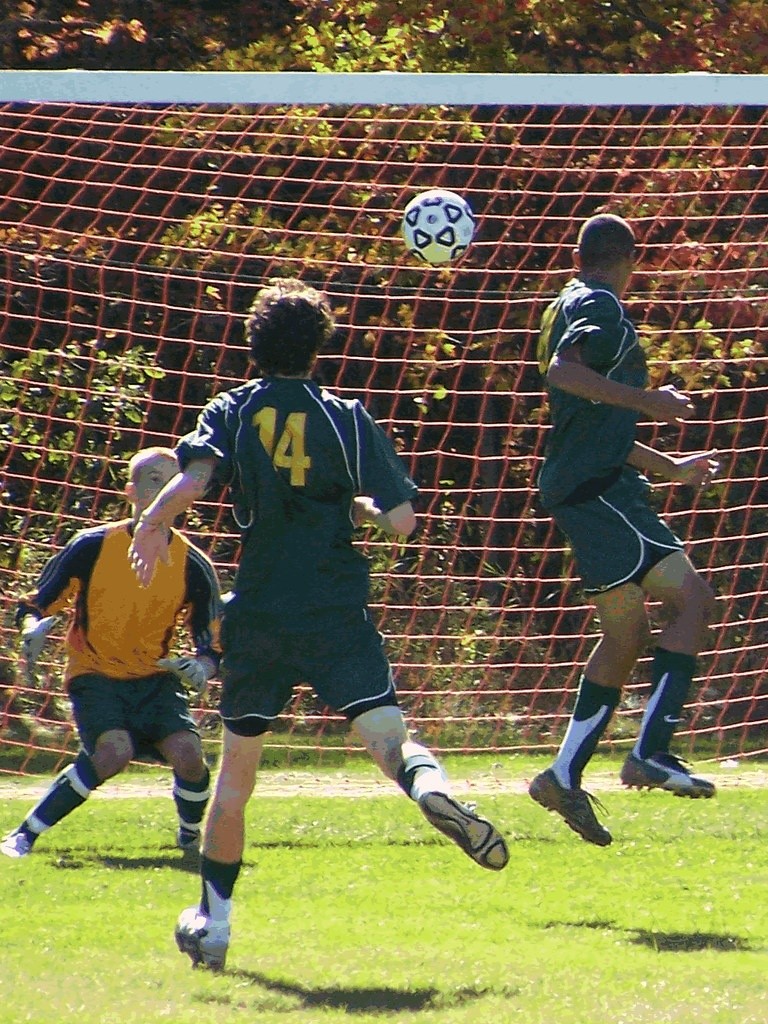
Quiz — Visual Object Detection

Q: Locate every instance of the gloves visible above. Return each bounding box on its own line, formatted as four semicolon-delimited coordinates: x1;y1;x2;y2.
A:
157;657;206;692
20;614;57;671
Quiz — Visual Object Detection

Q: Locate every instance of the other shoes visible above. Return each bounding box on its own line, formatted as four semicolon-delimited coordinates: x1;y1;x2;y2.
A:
179;825;199;849
0;830;30;859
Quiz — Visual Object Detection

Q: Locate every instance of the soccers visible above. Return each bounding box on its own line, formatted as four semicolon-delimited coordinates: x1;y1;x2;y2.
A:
400;188;475;264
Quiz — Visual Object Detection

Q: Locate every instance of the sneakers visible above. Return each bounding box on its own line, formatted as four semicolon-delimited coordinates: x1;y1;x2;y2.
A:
175;908;230;969
417;790;509;871
621;751;715;798
528;768;612;847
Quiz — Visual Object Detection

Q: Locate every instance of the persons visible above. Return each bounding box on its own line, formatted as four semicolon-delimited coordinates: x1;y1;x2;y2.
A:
0;447;225;860
528;211;719;847
129;276;508;971
